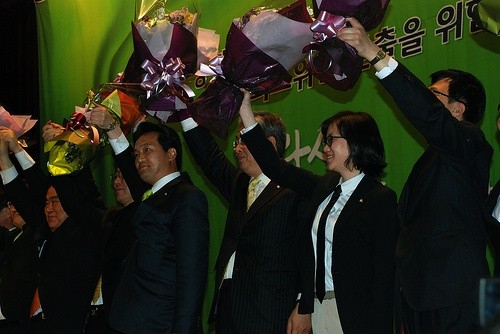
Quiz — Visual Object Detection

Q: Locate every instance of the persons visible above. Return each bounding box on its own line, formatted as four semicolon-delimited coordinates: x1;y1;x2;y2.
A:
0;129;54;334
44;104;156;334
178;110;316;334
337;17;500;334
239;87;398;334
1;125;108;334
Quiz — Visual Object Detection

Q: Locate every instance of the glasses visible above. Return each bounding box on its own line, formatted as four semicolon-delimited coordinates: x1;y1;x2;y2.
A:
7;202;12;208
430;88;467;108
109;172;124;180
321;135;345;147
232;138;244;148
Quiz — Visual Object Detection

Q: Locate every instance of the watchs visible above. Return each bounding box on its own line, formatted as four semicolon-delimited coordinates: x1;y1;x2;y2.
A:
369;48;387;66
105;118;118;132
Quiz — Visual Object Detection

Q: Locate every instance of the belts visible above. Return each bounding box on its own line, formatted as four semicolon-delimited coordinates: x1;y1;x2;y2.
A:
314;291;335;300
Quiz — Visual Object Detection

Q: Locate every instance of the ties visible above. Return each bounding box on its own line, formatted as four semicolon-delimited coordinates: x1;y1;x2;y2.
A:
218;178;262;289
315;185;343;305
93;274;102;304
142;189;153;202
31;287;40;317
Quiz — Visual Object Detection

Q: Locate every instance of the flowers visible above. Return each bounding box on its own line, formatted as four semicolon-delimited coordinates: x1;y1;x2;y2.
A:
136;1;202;31
41;84;114;164
234;0;316;30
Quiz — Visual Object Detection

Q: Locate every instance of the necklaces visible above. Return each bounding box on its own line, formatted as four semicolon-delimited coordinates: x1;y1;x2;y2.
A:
43;120;209;334
